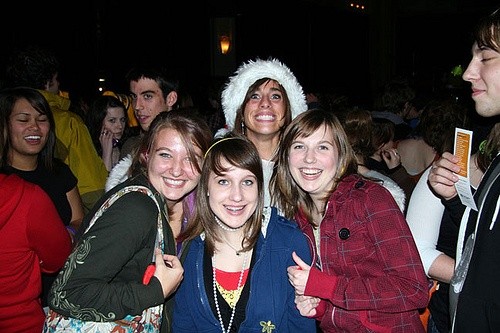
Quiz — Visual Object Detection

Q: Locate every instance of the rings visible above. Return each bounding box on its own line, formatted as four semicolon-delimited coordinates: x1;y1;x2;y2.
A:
394;152;398;155
102;131;108;136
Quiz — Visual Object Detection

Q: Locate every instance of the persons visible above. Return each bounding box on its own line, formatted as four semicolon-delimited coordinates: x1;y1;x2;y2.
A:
306;78;500;333
43;109;210;333
0;87;84;316
104;58;405;217
85;95;129;176
12;41;109;216
119;62;181;160
269;109;429;333
171;132;316;333
427;8;500;333
0;173;71;333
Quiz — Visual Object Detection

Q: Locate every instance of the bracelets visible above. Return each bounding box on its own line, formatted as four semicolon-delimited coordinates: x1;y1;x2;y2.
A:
66;226;77;243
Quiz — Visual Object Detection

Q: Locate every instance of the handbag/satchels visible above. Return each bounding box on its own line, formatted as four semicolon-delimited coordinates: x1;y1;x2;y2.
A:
41;185;164;332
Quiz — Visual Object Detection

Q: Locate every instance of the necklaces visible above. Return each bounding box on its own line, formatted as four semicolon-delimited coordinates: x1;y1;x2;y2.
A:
358;162;366;168
212;216;253;333
